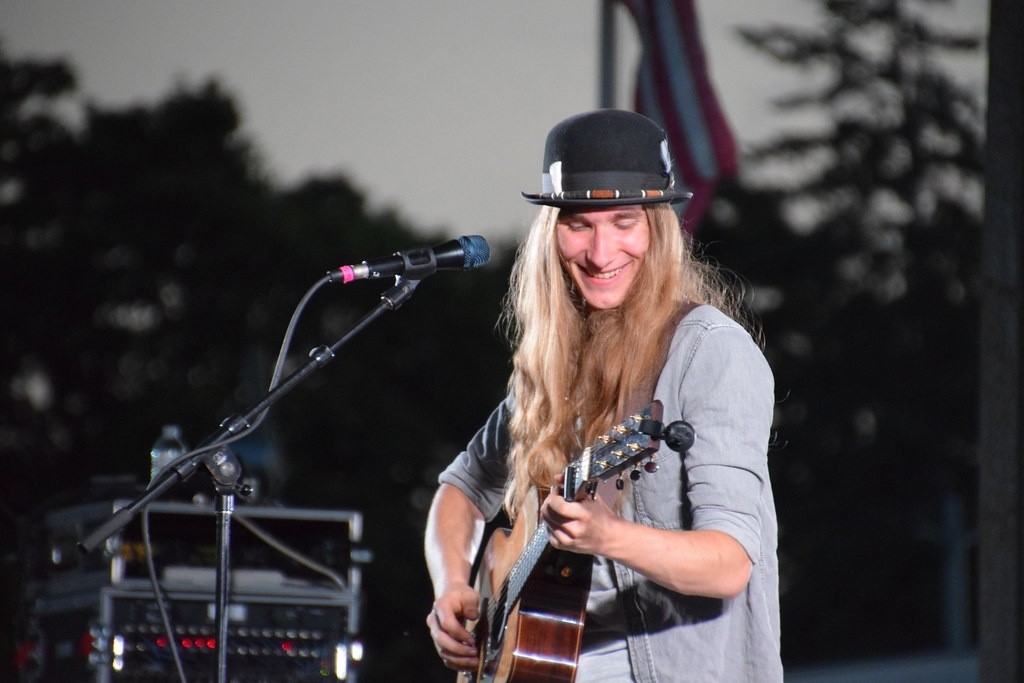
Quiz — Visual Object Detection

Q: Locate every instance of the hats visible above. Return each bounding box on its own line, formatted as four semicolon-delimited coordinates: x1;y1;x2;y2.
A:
520;109;694;209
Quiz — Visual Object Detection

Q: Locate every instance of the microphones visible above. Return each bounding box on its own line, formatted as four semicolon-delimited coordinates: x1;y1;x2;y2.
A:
327;236;491;283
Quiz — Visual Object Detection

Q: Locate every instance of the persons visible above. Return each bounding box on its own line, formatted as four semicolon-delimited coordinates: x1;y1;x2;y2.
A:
424;110;785;683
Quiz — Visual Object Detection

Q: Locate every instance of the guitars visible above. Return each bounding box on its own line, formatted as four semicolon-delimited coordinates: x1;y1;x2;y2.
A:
456;400;664;683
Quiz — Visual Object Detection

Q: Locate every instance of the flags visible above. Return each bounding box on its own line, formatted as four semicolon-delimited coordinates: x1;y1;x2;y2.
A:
621;1;739;227
